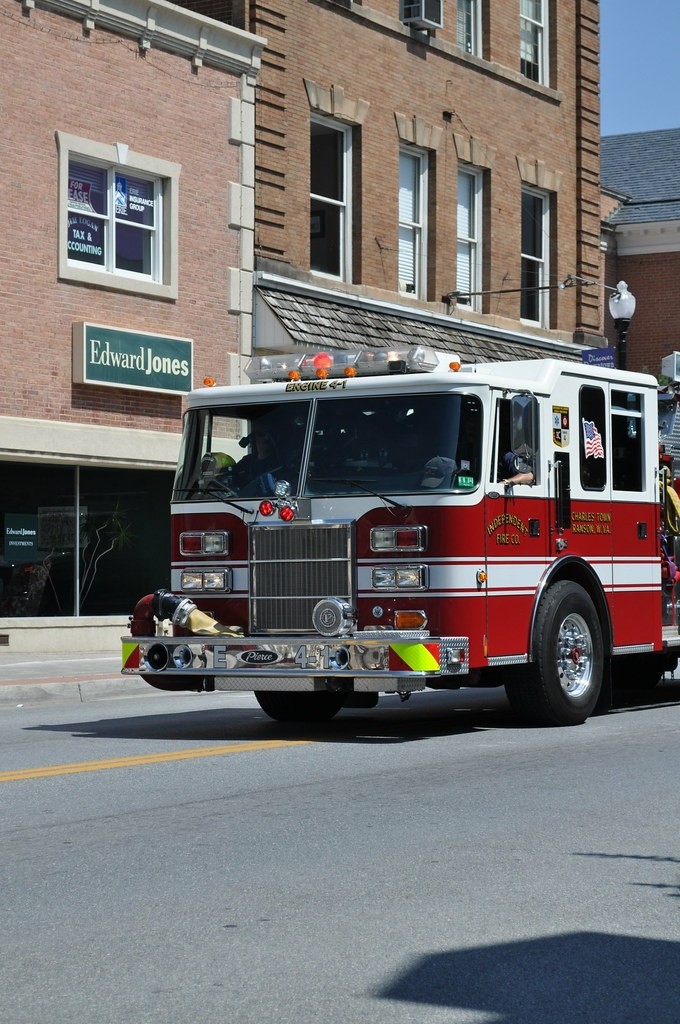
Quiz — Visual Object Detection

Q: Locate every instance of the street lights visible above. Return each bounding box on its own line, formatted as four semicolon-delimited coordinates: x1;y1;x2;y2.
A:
607;281;637;370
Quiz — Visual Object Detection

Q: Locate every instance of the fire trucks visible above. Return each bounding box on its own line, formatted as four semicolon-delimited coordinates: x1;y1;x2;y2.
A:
116;343;678;729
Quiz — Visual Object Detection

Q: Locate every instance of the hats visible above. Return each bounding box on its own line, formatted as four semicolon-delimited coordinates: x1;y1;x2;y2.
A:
420;456;457;489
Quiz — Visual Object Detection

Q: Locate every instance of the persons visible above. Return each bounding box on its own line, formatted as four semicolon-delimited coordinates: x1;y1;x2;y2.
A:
450;430;534;493
231;428;298;484
